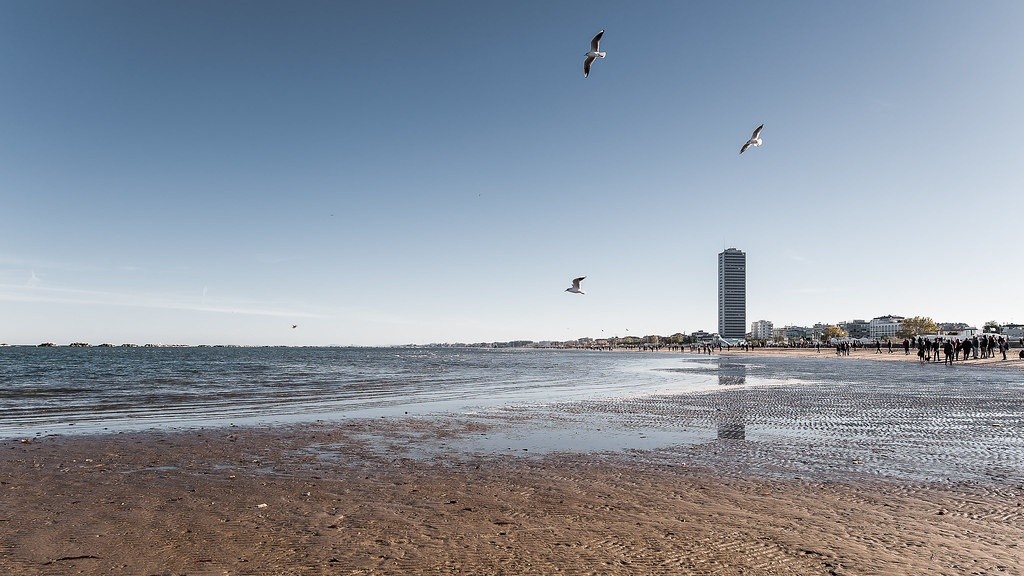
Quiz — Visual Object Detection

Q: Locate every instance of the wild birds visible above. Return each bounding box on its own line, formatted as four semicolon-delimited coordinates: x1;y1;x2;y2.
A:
564;276;587;295
738;124;765;156
292;325;298;328
583;29;607;79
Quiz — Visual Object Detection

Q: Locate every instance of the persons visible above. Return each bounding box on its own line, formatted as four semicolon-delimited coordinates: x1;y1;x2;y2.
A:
533;324;1024;368
